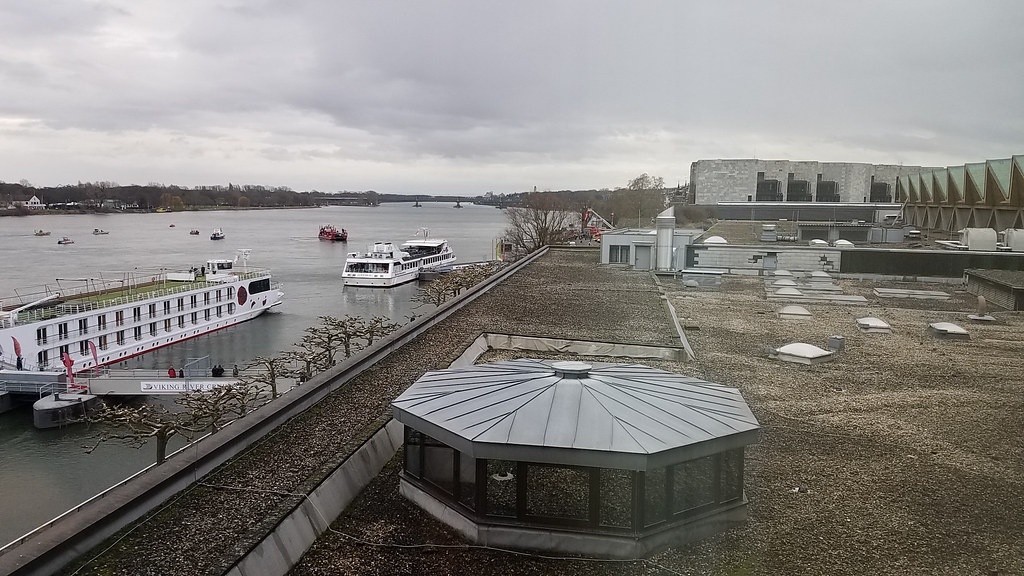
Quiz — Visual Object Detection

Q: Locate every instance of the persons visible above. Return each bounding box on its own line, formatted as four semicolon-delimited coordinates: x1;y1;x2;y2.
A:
445;247;448;252
189;267;193;273
348;263;369;272
17;354;23;371
232;365;239;377
212;365;224;377
201;265;205;276
40;361;44;371
179;368;184;378
168;366;176;378
0;352;5;370
575;235;593;246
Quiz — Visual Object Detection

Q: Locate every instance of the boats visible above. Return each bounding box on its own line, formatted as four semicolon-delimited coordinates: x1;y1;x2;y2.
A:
33;229;51;237
189;229;199;234
93;229;109;235
342;227;457;288
1;248;285;367
320;224;348;241
58;237;75;244
211;228;226;240
